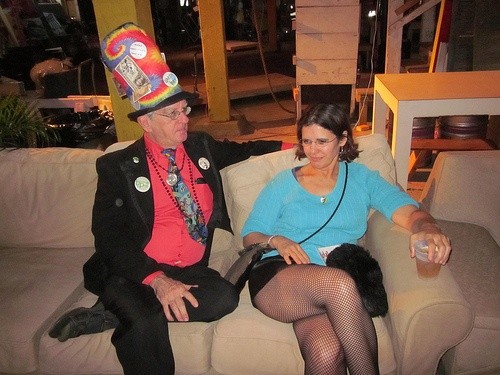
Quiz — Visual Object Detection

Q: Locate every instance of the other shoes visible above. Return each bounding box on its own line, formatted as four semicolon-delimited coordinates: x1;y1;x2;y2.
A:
48;296;114;339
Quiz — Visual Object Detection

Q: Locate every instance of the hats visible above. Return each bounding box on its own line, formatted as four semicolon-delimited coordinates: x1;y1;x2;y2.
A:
101;22;200;121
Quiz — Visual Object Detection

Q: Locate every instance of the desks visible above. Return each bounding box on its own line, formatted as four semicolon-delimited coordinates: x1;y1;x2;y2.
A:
372;69;500;191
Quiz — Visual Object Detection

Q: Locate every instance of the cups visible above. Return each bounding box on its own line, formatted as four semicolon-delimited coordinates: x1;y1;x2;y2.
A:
413;238;442;280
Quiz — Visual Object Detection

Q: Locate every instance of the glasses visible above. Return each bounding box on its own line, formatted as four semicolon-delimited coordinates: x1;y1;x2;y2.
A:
154;107;191;120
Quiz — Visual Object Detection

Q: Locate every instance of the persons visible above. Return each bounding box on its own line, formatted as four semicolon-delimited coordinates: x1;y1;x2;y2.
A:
240;101;452;375
47;68;301;375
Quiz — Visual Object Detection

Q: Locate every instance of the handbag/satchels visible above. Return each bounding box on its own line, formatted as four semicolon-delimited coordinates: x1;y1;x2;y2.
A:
224;243;270;296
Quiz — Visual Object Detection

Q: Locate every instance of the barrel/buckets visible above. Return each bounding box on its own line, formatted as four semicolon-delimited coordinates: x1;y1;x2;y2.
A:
438;115;488;151
386;109;436;167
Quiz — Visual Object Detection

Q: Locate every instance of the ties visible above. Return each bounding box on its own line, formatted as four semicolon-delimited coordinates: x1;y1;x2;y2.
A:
161;148;208;247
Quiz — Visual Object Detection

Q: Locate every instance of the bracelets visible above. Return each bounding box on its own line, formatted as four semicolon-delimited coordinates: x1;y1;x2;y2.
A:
268;235;279;249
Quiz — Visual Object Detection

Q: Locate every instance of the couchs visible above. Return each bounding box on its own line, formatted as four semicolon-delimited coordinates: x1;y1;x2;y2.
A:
0;133;473;375
420;152;500;375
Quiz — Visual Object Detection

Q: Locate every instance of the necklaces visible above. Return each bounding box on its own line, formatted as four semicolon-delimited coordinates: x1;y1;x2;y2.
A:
301;164;339;204
145;147;205;232
